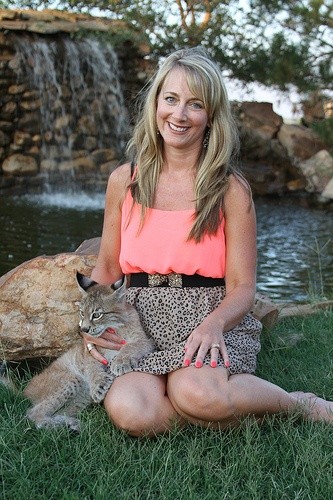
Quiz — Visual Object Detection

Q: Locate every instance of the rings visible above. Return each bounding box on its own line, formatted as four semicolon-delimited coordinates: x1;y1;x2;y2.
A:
210;344;221;351
86;344;96;354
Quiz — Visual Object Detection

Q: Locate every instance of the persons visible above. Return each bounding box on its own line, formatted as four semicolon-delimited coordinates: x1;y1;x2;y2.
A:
79;46;333;436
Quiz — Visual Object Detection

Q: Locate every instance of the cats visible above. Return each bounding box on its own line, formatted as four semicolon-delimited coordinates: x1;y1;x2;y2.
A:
23;272;153;434
